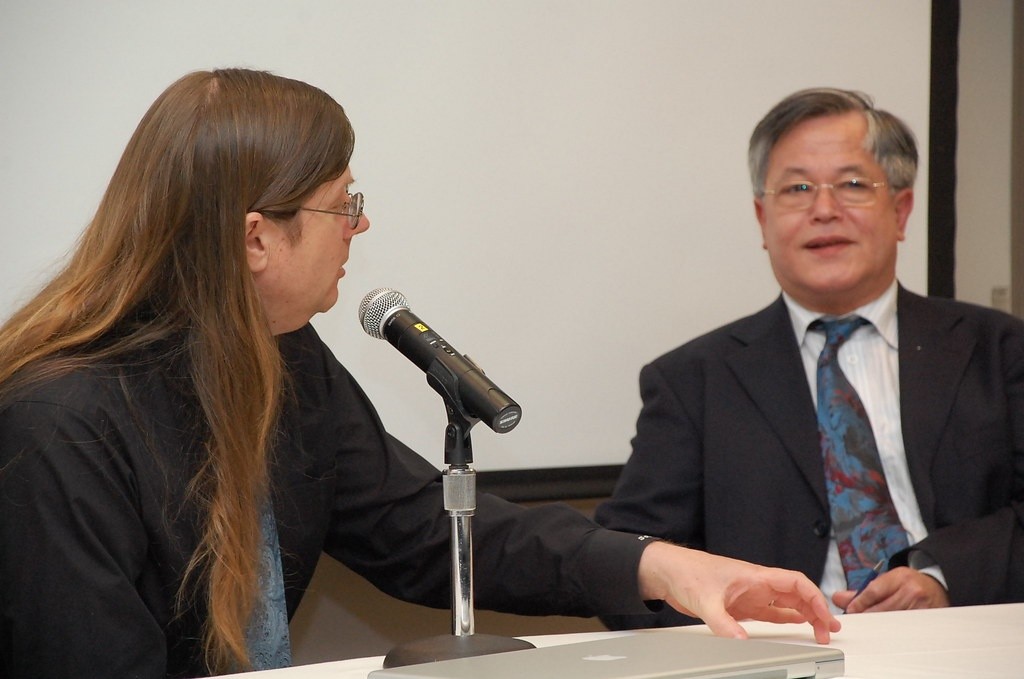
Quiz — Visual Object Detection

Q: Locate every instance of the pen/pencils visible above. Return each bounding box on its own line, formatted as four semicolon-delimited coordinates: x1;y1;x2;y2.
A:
843;558;885;615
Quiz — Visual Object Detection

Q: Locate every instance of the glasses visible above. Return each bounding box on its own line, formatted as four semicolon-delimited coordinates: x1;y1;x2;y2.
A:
298;192;364;229
761;175;892;211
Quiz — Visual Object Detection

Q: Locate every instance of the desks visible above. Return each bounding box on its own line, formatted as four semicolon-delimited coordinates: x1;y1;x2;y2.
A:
202;603;1023;679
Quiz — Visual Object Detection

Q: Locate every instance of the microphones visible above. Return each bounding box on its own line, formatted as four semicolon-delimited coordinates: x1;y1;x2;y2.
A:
359;286;522;434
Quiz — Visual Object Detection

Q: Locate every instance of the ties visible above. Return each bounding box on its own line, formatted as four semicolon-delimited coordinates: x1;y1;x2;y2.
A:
807;316;909;589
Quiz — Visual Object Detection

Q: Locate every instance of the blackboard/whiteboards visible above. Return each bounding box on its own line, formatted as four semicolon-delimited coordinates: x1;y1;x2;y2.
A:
0;0;958;502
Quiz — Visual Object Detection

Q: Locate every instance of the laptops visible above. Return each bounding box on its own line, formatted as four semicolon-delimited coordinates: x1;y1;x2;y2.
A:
365;630;845;679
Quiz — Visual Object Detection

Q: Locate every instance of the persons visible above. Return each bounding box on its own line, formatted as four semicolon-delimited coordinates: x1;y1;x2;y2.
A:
0;69;841;679
593;85;1022;629
